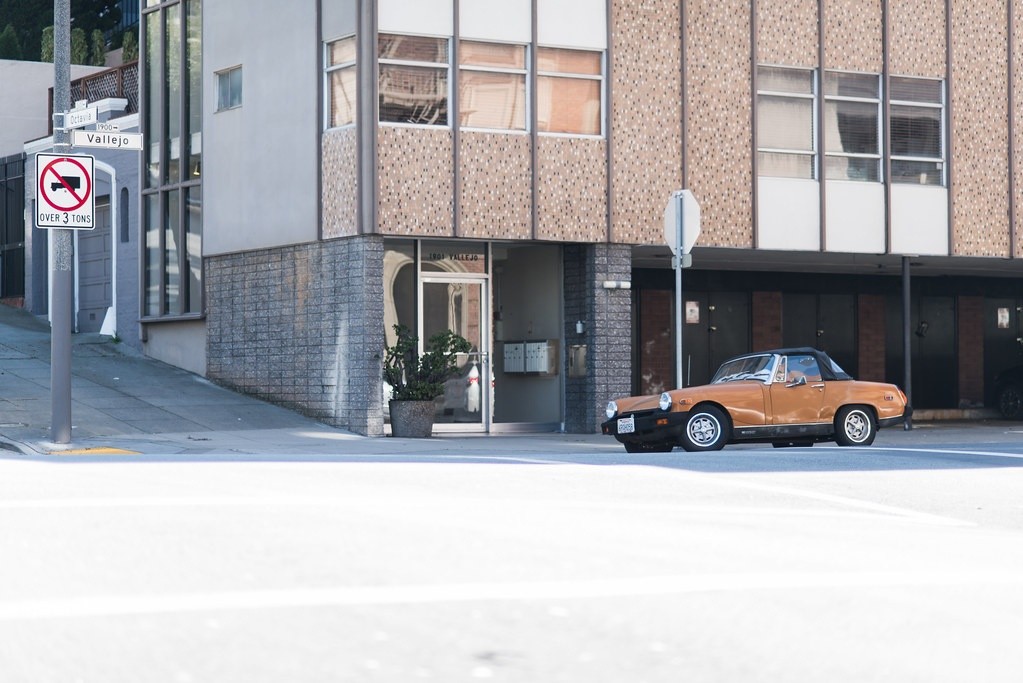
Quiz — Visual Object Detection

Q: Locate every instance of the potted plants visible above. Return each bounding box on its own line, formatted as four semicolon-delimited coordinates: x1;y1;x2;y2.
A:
384;325;472;438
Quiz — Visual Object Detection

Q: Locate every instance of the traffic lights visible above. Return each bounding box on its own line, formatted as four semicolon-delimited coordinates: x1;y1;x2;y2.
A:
94;0;122;33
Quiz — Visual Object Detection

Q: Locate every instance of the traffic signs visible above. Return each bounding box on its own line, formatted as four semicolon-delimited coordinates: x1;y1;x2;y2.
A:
71;123;144;150
64;106;98;129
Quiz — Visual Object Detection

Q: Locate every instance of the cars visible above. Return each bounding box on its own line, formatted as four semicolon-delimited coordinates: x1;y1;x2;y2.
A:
600;347;913;454
